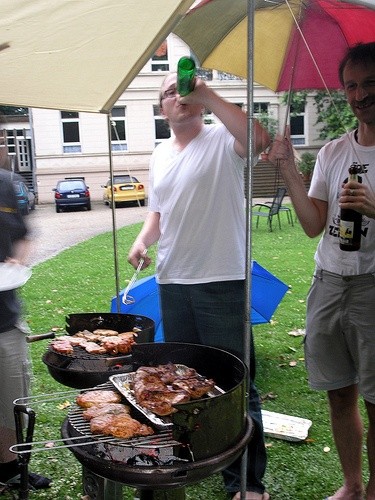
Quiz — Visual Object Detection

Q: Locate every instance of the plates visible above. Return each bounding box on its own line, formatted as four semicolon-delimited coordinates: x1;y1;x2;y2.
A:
0;262;32;291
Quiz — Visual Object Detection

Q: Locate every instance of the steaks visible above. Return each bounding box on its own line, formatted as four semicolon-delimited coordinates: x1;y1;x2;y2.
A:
131;365;215;416
50;328;135;354
76;391;154;438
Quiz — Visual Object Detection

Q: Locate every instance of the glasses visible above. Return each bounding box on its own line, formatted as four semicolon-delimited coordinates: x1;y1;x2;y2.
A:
162;88;177;99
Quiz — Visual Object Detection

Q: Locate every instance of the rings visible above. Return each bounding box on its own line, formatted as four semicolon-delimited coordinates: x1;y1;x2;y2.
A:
350;189;354;195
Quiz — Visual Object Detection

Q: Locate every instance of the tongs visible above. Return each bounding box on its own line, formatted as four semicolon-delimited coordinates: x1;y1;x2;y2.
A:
122;249;148;305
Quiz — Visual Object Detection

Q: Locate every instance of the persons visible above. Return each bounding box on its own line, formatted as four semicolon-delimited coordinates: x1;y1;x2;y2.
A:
0;167;51;491
128;73;276;500
266;41;375;500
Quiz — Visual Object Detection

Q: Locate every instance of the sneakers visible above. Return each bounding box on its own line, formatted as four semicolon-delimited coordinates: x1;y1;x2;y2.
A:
0;469;49;493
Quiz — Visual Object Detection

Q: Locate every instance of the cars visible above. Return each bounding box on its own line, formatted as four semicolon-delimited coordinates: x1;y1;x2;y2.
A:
52;179;92;212
100;175;146;208
12;180;36;214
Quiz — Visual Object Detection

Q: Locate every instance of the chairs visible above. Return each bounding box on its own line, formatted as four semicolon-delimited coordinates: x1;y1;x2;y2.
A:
252;188;287;232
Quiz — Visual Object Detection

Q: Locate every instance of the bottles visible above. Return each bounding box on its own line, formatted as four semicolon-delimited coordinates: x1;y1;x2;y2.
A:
176;56;196;105
339;164;362;252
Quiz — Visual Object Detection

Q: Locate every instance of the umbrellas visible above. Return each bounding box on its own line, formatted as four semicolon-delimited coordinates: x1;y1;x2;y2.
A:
110;260;292;343
174;0;375;138
